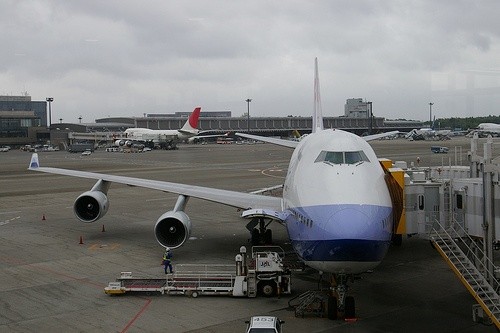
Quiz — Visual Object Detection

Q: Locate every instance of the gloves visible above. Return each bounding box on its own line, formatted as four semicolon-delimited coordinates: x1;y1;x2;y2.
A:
168;259;170;261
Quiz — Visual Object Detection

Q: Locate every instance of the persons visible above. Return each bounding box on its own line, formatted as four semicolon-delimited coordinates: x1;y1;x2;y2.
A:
162;248;174;274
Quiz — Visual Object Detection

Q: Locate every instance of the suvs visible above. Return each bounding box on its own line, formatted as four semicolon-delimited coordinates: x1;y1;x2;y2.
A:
244;316;285;333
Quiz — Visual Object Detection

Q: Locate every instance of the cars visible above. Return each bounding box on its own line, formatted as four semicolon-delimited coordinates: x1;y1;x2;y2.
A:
82;151;92;156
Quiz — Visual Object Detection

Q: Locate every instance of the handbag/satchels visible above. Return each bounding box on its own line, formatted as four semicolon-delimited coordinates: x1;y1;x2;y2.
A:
161;260;164;265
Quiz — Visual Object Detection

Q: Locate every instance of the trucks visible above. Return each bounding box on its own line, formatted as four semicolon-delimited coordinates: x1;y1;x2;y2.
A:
104;246;292;298
431;146;449;153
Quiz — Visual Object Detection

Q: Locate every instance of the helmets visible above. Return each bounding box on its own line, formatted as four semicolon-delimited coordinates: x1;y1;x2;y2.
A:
166;247;170;251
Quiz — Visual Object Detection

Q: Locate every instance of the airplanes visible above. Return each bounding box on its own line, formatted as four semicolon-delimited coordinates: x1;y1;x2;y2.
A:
27;57;399;320
115;107;201;150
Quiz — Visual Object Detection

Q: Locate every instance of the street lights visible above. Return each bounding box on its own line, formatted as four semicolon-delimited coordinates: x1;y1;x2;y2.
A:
46;97;54;128
245;99;252;131
